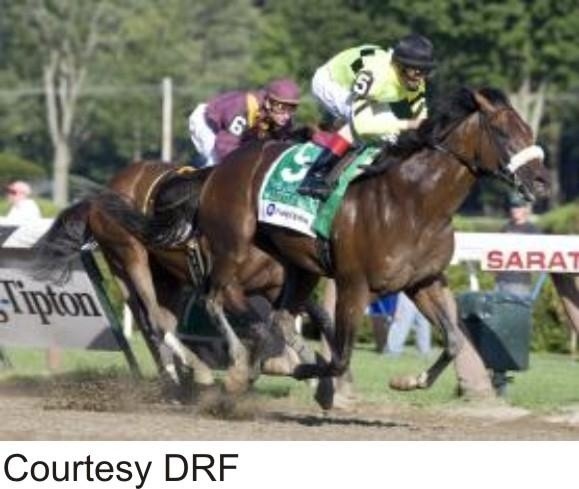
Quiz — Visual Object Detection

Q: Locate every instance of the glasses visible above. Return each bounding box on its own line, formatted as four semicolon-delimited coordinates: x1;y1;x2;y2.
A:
269;99;298;114
397;63;436;82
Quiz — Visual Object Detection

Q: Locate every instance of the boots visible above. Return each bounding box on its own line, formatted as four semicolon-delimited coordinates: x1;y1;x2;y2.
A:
296;130;354;202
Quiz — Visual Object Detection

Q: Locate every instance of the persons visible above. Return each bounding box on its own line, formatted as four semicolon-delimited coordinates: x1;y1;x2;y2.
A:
369;293;398;352
6;181;41;220
494;186;543;298
187;76;302;169
297;35;438;196
383;291;432;354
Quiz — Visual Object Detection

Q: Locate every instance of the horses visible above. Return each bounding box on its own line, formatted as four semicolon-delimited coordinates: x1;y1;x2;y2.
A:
68;82;553;416
21;116;363;410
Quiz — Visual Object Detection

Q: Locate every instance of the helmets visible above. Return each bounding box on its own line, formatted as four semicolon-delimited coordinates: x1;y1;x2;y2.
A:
266;80;300;106
392;33;437;70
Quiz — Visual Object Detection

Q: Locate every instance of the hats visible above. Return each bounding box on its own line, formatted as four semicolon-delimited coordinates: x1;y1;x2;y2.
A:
3;180;31;198
